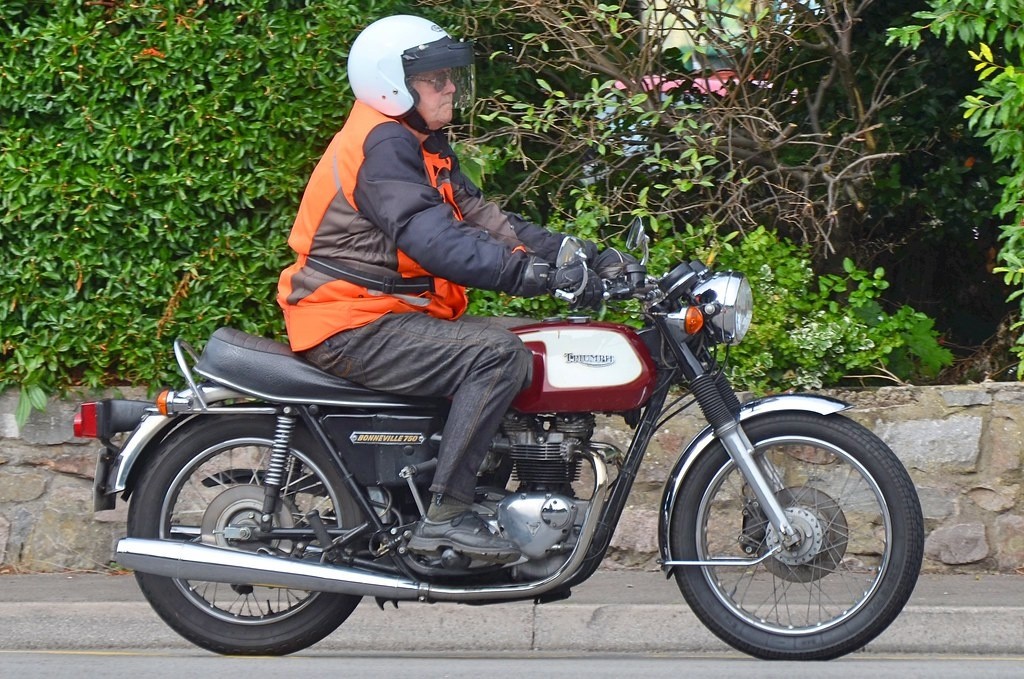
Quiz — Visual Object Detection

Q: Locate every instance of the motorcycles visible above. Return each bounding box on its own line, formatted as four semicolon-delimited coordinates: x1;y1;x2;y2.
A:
72;215;924;661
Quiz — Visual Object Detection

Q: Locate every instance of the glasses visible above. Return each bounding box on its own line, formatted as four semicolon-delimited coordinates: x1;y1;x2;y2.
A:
406;69;461;92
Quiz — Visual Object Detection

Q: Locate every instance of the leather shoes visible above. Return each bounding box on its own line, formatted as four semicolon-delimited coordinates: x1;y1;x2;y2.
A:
406;509;525;564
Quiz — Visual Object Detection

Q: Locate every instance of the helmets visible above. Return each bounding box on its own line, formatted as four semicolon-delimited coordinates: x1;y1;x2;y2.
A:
347;15;477;133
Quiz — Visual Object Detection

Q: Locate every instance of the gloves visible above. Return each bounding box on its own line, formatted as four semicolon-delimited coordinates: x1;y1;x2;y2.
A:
586;243;638;301
521;253;607;313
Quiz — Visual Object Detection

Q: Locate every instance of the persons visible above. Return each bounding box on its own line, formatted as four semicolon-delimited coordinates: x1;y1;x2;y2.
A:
277;15;637;563
